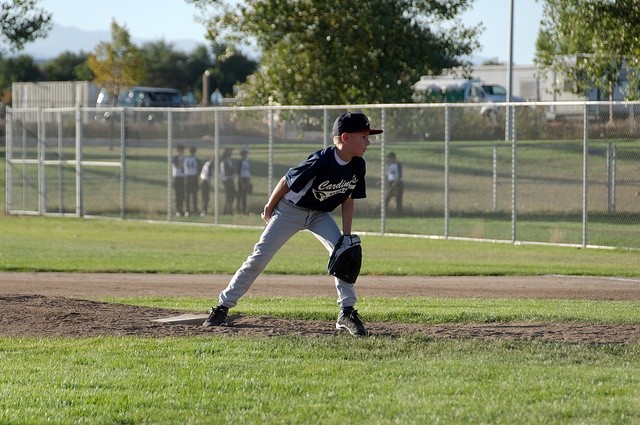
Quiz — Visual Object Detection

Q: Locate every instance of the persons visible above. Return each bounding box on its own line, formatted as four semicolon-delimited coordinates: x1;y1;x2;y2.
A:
200;157;215;216
224;146;233;168
182;146;203;218
237;148;251;215
172;143;185;217
384;152;403;216
203;113;384;338
220;152;235;215
183;87;224;131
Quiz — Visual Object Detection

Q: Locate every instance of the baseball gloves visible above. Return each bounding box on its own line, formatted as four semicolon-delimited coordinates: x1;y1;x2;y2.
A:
328;234;362;284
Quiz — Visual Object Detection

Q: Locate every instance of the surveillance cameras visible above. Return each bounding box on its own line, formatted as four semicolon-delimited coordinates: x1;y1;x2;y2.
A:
480;103;503;135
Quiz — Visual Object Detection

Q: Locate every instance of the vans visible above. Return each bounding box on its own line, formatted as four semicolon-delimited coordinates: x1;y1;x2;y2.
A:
94;86;190;122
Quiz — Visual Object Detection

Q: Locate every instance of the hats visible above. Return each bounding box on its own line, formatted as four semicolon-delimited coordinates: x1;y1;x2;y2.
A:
332;112;383;135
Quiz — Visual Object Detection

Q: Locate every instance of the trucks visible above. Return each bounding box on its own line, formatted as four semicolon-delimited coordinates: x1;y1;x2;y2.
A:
412;75;526;114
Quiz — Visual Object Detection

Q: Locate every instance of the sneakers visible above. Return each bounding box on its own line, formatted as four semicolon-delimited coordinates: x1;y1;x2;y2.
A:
336;309;366;337
202;305;229;327
